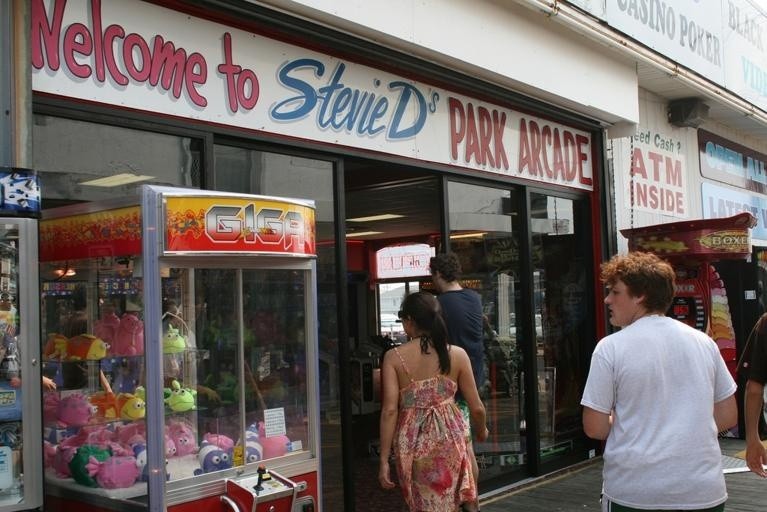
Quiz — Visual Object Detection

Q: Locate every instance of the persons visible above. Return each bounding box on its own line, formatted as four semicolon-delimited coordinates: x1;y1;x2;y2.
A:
62;282;113;393
378;290;490;511
735;312;767;478
157;298;219;403
579;251;739;511
429;254;485;511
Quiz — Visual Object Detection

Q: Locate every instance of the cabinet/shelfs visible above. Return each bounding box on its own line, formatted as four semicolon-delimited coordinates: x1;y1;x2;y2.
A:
35;183;321;512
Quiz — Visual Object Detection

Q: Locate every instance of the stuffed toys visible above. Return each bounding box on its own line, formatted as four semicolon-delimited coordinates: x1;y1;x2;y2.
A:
161;325;290;480
42;309;148;490
42;309;290;490
